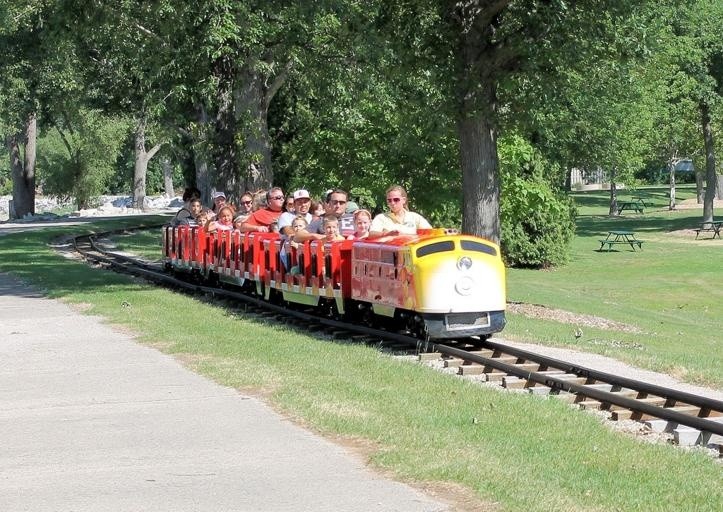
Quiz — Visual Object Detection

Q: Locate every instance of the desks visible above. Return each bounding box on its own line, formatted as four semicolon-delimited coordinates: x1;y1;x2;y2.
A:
600;231;643;252
617;197;649;215
695;221;723;239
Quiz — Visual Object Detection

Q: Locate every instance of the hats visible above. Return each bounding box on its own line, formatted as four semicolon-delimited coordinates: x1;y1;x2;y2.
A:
213;192;226;200
293;189;311;203
345;201;359;214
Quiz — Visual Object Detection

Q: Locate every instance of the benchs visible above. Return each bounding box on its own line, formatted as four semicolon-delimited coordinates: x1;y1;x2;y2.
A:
598;240;644;244
693;229;722;232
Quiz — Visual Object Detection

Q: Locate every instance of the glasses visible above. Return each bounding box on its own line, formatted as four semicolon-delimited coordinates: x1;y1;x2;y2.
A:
330;200;346;205
269;195;285;200
386;197;407;203
241;200;251;205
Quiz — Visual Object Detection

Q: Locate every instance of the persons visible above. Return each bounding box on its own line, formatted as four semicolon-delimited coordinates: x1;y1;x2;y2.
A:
167;182;435;278
